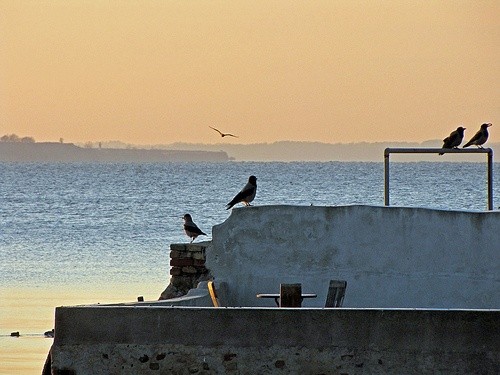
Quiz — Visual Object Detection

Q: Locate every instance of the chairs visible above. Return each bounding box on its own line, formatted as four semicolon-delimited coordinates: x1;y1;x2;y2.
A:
207;281;221;306
280;283;303;307
325;279;348;307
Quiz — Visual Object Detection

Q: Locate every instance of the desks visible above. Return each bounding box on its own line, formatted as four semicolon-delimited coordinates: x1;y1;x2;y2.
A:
256;294;318;307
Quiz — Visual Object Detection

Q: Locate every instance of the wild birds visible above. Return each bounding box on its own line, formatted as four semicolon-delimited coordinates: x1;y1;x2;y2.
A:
208;125;240;139
438;127;467;155
463;123;492;150
181;214;208;244
225;175;259;210
